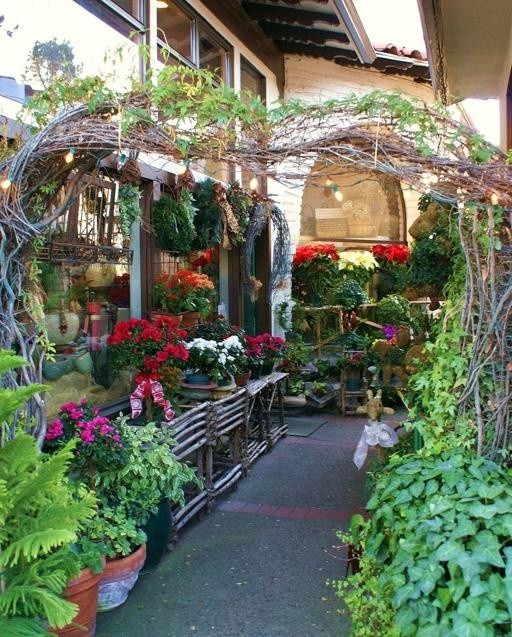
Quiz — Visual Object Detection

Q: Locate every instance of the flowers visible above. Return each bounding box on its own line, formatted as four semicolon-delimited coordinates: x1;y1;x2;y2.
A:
45;397;131;490
105;243;277;375
277;242;412;377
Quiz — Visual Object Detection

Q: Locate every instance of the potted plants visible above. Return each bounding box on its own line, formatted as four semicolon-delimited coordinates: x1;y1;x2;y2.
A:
95;422;206;572
97;501;147;612
45;548;106;637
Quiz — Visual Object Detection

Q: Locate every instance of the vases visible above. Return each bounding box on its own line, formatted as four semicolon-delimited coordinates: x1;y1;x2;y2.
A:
235;369;252;386
41;310;108;380
213;382;236;400
186;375;209;386
